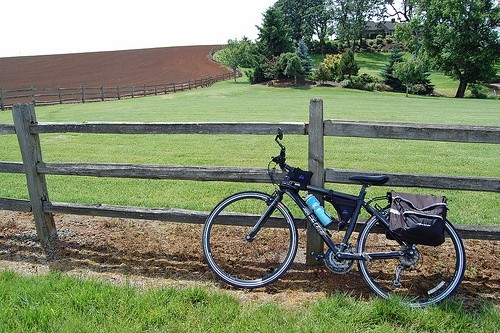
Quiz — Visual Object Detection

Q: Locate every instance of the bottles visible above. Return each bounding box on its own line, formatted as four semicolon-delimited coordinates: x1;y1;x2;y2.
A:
302;193;335;227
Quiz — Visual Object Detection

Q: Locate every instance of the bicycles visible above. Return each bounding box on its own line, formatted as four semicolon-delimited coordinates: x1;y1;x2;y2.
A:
201;127;467;310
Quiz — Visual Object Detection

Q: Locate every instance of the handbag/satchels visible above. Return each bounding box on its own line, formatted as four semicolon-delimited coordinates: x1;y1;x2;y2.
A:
386;190;447;247
